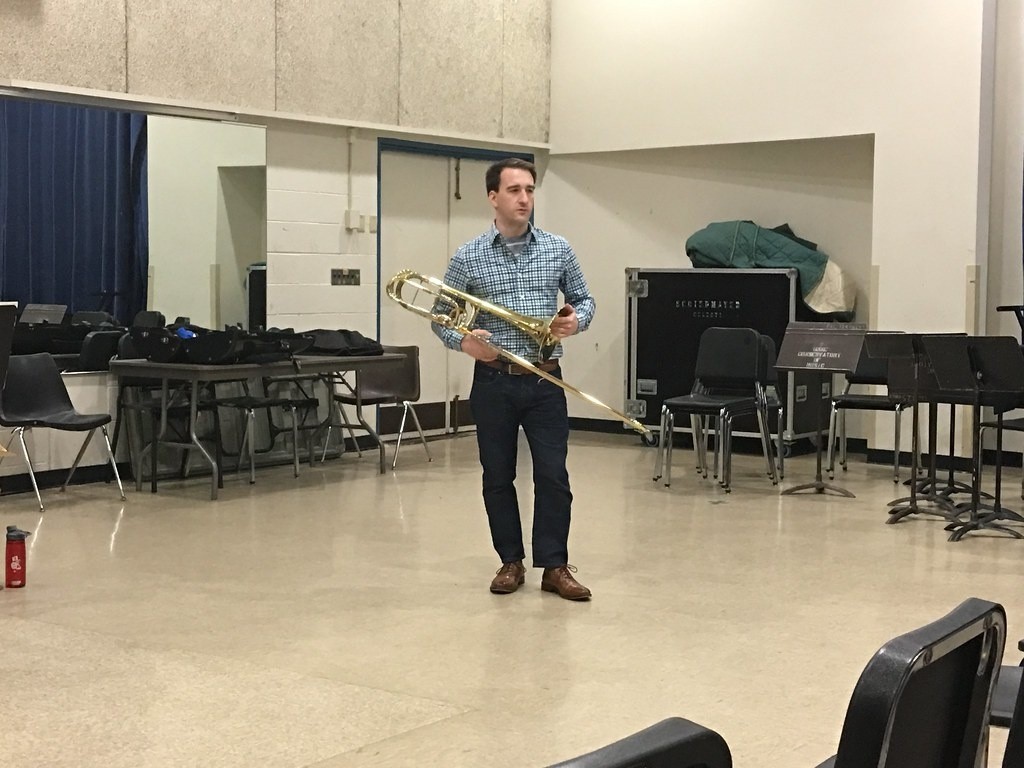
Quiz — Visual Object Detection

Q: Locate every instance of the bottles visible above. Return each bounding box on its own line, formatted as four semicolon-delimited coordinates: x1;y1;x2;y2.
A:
5;525;27;588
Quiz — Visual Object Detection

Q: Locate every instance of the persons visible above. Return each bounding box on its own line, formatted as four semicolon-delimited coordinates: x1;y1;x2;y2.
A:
431;158;596;599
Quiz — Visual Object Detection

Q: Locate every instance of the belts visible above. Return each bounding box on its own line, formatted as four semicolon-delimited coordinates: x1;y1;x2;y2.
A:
488;358;559;375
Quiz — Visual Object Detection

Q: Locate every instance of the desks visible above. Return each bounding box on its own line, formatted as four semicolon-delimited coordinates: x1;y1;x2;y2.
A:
109;350;407;500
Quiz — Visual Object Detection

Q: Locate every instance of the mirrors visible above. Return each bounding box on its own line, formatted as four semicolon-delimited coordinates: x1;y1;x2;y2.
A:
1;90;267;376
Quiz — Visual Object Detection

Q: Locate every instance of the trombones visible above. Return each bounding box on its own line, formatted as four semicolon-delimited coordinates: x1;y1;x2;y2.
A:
385;268;651;436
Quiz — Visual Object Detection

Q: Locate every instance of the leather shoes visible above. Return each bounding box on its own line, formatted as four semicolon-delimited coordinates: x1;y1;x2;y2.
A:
541;564;592;600
490;561;526;593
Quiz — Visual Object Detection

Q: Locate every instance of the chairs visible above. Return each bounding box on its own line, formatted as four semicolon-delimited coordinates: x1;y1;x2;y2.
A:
1;298;265;373
105;377;318;500
0;352;127;512
322;345;434;467
653;328;1024;540
531;598;1024;767
